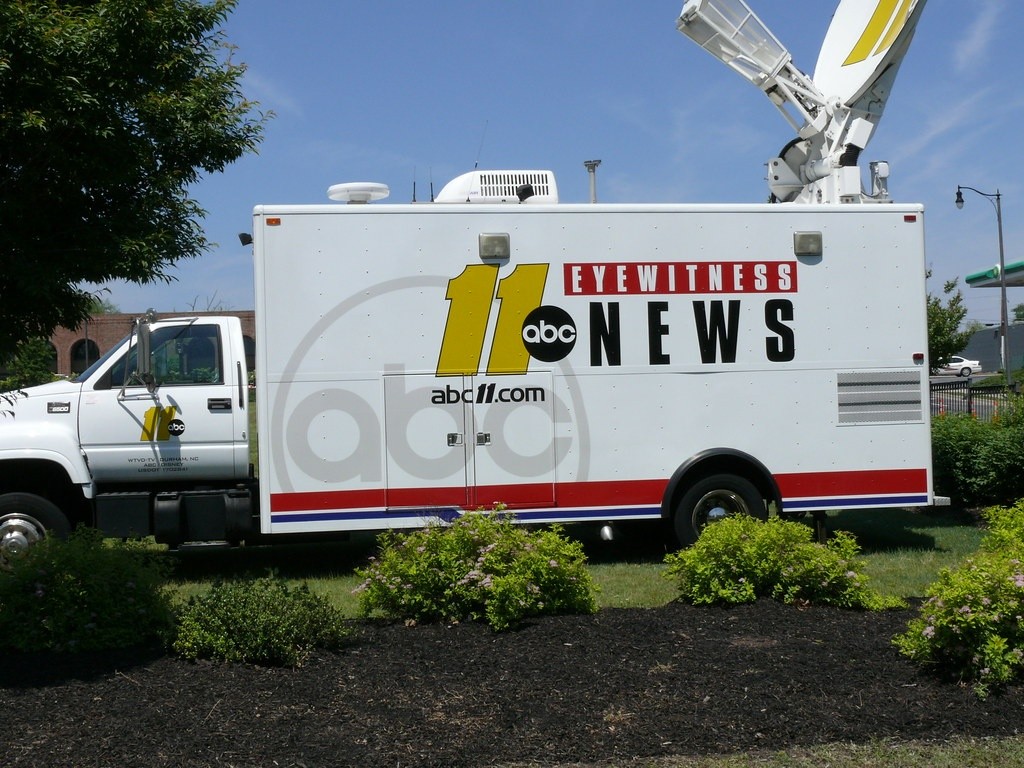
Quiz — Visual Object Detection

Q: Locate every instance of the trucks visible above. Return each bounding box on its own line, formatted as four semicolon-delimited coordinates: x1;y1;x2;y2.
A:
0;167;952;570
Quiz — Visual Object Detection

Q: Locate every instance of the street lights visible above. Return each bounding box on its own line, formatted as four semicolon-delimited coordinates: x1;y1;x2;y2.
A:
954;184;1014;386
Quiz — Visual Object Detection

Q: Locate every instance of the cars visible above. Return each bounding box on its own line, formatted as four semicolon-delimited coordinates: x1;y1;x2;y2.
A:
930;352;982;377
